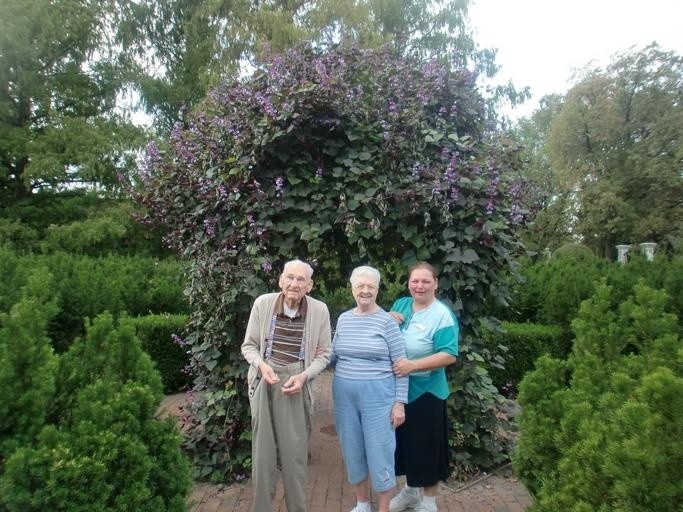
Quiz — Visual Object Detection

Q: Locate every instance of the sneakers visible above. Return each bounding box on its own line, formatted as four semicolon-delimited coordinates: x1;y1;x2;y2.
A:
415;501;438;512
351;501;371;512
389;488;422;512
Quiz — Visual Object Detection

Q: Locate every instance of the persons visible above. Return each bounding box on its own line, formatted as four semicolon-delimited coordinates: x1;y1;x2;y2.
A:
387;262;458;512
240;260;332;512
325;266;409;512
